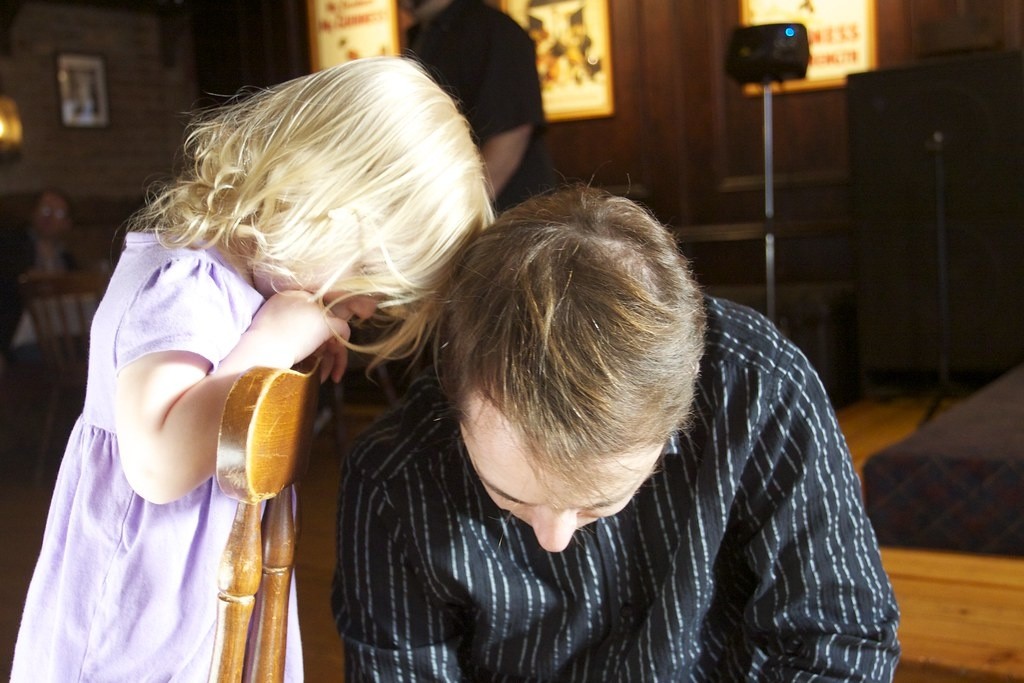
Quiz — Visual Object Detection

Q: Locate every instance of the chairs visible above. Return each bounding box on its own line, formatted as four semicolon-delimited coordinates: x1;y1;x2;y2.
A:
217;356;322;683
17;270;109;488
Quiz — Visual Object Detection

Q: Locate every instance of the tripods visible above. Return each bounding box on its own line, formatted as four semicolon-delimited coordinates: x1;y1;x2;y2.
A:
877;134;967;431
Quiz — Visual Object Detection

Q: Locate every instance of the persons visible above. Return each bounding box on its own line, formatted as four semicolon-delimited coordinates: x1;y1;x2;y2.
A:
397;0;552;213
329;185;902;683
0;185;90;386
13;54;494;683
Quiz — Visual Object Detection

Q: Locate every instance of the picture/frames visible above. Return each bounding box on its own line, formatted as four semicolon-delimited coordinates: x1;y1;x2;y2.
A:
55;48;113;132
